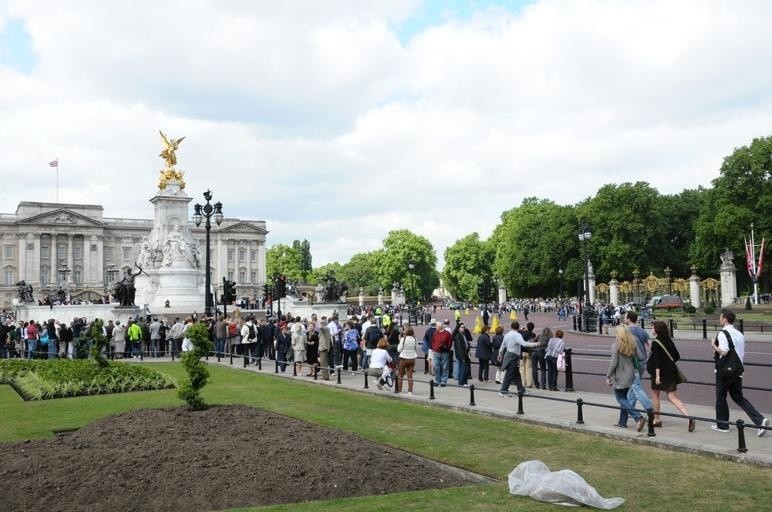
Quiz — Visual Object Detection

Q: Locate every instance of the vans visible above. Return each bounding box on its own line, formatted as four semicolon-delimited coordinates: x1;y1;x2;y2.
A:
646;294;683;311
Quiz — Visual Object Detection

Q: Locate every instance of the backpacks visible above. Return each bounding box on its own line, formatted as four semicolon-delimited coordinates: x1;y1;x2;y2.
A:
245;322;255;340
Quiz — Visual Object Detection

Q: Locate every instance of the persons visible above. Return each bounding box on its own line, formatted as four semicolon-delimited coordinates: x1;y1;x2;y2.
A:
20;281;33;301
57;287;66;305
647;321;695;432
624;310;652;418
325;270;339;301
164;133;177;167
711;310;769;437
605;325;646;432
120;268;141;306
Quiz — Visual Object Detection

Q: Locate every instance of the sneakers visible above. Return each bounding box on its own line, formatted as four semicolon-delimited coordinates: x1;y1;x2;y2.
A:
710;423;730;433
613;417;662;432
756;417;770;438
688;417;696;433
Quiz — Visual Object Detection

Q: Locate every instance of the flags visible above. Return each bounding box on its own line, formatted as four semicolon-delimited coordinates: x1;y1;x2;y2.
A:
49;161;58;167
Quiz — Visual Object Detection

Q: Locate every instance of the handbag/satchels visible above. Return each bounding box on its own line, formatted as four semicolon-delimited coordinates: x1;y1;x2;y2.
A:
715;350;745;389
676;365;687;385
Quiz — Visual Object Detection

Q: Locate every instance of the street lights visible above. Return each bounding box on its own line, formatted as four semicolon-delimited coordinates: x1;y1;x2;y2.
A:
688;262;702;311
192;189;224;317
558;264;564;300
609;269;620;305
106;262;119;284
407;259;418;311
630;269;641;299
577;219;597;311
663;265;672;295
57;262;72;281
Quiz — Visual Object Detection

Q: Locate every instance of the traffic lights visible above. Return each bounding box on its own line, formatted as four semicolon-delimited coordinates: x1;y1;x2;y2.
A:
277;273;287;298
225;280;237;305
261;284;268;301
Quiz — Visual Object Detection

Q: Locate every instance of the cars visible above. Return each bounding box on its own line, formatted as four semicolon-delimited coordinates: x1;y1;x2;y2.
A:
445;296;483;310
614;301;645;313
